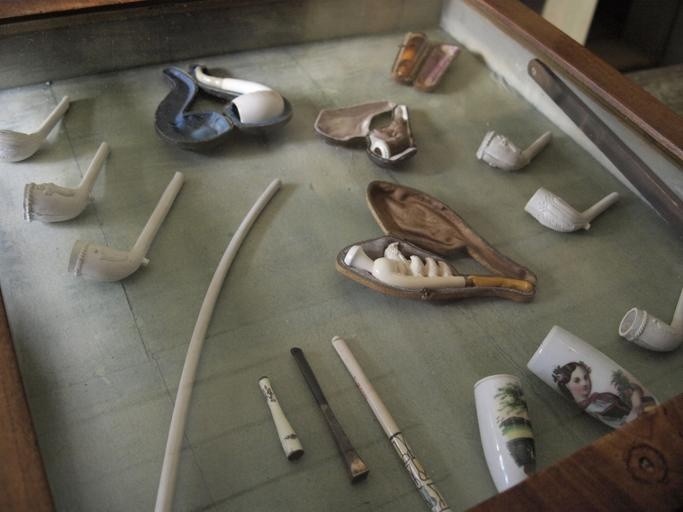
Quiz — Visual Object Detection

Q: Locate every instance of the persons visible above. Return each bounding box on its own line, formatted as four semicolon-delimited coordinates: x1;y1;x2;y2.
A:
553;361;655;429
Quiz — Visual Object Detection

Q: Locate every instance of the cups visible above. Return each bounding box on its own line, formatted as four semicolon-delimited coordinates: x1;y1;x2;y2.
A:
523;324;659;430
472;374;536;493
